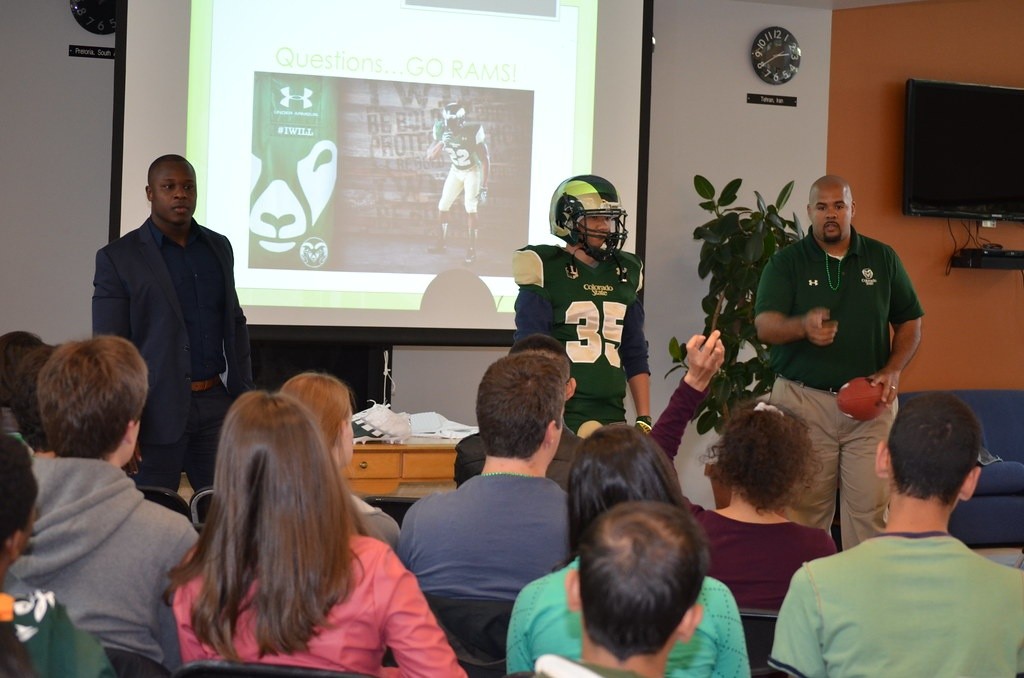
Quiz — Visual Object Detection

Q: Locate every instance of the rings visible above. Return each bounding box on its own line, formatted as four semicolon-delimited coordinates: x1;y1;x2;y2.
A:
891;386;895;389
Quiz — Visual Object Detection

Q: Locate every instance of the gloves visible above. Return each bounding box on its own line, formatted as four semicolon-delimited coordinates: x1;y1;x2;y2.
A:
474;187;488;209
441;129;453;150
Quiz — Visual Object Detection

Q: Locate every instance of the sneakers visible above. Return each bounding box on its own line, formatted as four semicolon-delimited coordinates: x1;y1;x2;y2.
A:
352;399;412;445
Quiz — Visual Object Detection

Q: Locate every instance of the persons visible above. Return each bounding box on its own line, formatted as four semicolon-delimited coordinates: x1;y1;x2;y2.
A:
277;372;401;548
651;329;840;610
454;332;586;490
427;102;490;260
504;498;711;678
768;389;1024;678
509;173;653;440
92;154;254;522
755;173;924;551
504;426;750;678
0;330;200;678
174;389;468;678
390;352;571;601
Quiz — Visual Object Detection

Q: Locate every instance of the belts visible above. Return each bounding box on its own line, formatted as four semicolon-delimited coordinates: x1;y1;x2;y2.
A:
192;375;220;392
777;372;839;393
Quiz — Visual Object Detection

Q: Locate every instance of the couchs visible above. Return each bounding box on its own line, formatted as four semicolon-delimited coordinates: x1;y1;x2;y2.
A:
895;390;1024;546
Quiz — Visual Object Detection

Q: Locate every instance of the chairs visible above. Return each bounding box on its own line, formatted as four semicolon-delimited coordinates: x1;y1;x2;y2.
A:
106;486;789;678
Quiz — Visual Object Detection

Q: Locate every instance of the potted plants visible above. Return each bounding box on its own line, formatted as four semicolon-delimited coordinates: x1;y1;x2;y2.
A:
663;174;807;510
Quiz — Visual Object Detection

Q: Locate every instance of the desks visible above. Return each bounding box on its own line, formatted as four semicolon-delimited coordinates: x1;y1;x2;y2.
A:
341;444;457;496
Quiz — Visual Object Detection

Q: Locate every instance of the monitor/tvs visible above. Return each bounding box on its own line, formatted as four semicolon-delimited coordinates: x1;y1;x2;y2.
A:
901;78;1024;223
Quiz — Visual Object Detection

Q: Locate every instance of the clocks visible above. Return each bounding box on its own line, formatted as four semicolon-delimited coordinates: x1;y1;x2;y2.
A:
751;27;802;86
69;0;115;36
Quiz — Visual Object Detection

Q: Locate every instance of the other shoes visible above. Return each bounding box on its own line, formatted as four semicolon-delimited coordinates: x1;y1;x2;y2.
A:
427;244;448;255
464;248;476;263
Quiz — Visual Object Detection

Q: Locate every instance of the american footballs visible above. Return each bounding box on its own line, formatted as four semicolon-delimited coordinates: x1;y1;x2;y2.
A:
836;376;886;421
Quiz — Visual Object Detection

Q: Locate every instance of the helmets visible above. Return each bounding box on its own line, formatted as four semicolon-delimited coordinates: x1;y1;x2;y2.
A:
442;102;466;137
548;174;623;246
443;103;468;132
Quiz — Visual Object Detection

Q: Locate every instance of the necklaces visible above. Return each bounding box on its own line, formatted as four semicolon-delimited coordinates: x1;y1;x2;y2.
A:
823;244;848;291
481;467;537;479
